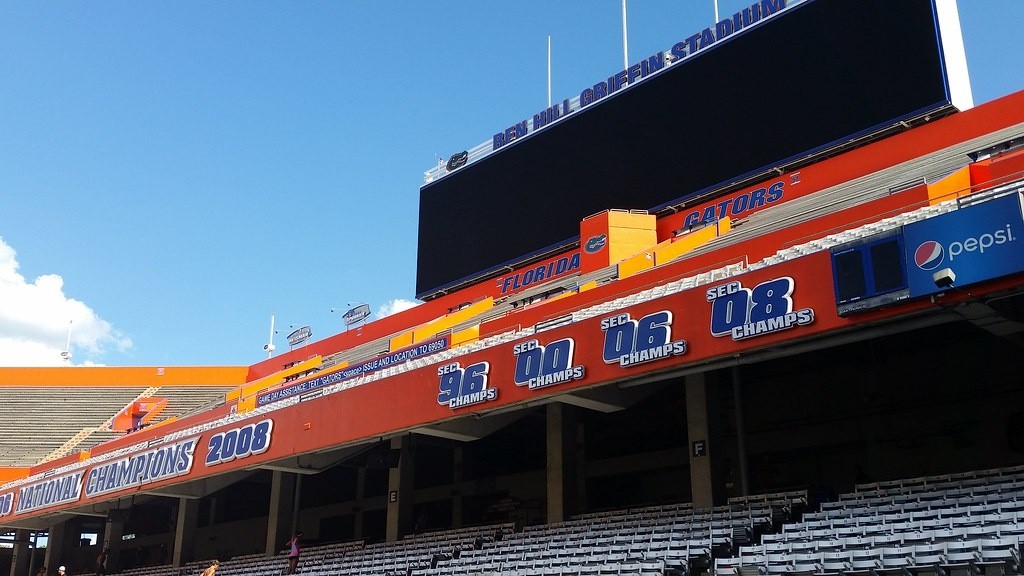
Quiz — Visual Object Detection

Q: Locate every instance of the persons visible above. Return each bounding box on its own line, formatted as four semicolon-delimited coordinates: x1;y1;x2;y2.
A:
96;549;110;576
58;566;67;576
199;560;220;576
286;532;303;575
35;567;47;576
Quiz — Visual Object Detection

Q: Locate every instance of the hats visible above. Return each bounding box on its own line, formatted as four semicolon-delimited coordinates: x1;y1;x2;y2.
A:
59;566;66;571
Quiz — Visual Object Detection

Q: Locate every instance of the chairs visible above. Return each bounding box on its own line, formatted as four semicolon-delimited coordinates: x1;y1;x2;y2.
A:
121;467;1024;576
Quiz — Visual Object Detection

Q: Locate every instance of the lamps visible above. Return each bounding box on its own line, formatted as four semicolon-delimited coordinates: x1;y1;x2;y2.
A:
376;437;402;469
104;498;129;522
932;268;956;289
